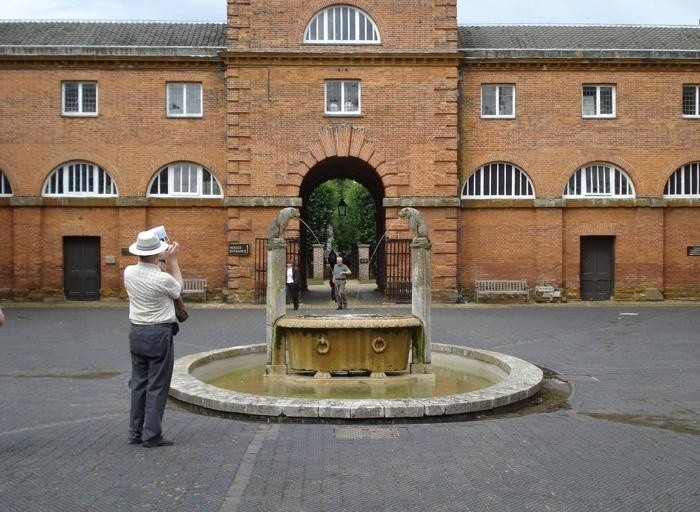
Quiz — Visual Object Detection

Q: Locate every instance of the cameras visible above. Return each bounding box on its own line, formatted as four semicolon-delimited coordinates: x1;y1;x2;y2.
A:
164;245;173;252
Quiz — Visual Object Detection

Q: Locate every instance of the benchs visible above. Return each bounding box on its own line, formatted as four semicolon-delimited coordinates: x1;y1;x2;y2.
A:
182;278;208;302
474;279;530;303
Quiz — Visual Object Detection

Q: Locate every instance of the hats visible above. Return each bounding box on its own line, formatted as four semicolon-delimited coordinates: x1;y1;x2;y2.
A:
128;231;169;257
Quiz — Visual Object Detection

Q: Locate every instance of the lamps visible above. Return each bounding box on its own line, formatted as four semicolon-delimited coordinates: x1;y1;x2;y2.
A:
336;177;348;217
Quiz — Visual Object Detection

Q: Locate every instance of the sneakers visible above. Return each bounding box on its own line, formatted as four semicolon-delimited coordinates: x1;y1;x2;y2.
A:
131;437;174;447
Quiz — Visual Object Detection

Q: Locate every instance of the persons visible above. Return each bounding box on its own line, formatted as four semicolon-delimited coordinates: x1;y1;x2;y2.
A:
121;228;187;449
330;256;352;310
283;259;300;311
